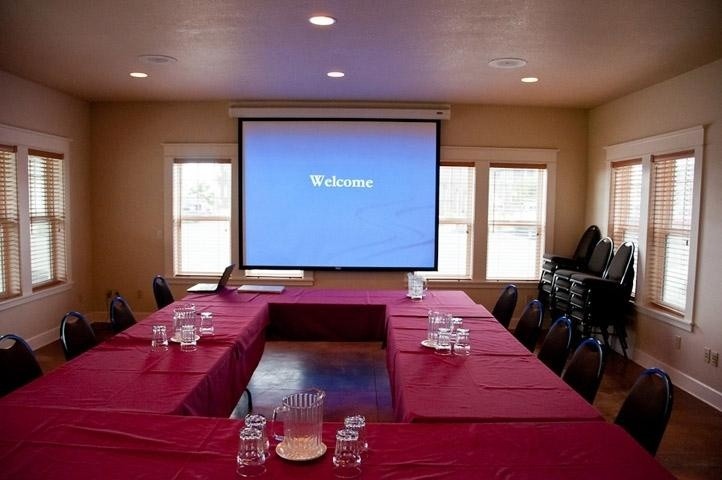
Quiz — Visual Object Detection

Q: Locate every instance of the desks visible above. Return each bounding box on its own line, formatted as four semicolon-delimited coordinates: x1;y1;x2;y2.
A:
0;409;678;480
182;286;475;337
382;303;606;423
1;301;270;415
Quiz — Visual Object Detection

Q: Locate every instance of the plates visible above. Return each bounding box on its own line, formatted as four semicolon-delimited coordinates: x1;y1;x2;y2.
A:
171;334;200;342
276;438;328;461
420;339;451;349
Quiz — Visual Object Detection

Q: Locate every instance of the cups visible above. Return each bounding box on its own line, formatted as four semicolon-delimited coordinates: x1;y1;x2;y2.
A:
343;415;368;451
454;328;471;356
435;328;451;355
451;317;464;333
151;324;169;351
333;429;361;468
245;413;270;450
272;405;286;442
180;325;197;352
200;312;216;337
236;427;267;478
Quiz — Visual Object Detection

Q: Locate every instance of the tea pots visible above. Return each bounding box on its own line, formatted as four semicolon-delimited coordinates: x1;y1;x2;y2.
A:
275;390;328;461
428;310;454;346
407;273;428;296
172;302;197;340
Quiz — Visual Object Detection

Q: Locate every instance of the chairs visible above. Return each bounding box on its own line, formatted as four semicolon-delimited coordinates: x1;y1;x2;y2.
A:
554;237;614;315
562;337;606;405
110;297;138;334
537;316;573;378
0;334;43;398
492;284;518;329
569;240;635;361
613;368;674;458
539;224;601;311
153;275;175;309
513;299;544;354
60;311;96;361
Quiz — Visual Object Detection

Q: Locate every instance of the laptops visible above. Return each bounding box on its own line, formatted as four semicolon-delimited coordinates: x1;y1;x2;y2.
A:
186;263;236;292
237;284;286;294
